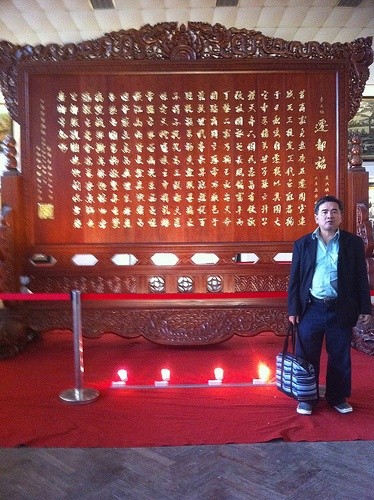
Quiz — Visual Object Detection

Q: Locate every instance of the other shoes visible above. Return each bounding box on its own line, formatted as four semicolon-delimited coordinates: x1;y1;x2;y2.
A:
296;400;312;415
333;402;354;414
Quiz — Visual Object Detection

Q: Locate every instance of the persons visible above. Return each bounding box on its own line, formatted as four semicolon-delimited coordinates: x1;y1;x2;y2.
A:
285;195;373;415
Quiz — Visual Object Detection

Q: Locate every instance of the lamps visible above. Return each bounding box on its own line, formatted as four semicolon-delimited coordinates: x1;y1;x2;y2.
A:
153;369;171;387
110;370;129;389
208;367;224;386
251;362;275;385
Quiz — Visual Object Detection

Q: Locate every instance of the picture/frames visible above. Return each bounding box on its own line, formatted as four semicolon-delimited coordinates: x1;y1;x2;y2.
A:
347;95;374;164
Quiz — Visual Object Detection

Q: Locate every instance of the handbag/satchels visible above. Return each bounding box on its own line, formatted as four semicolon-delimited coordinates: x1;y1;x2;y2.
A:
275;321;319;403
